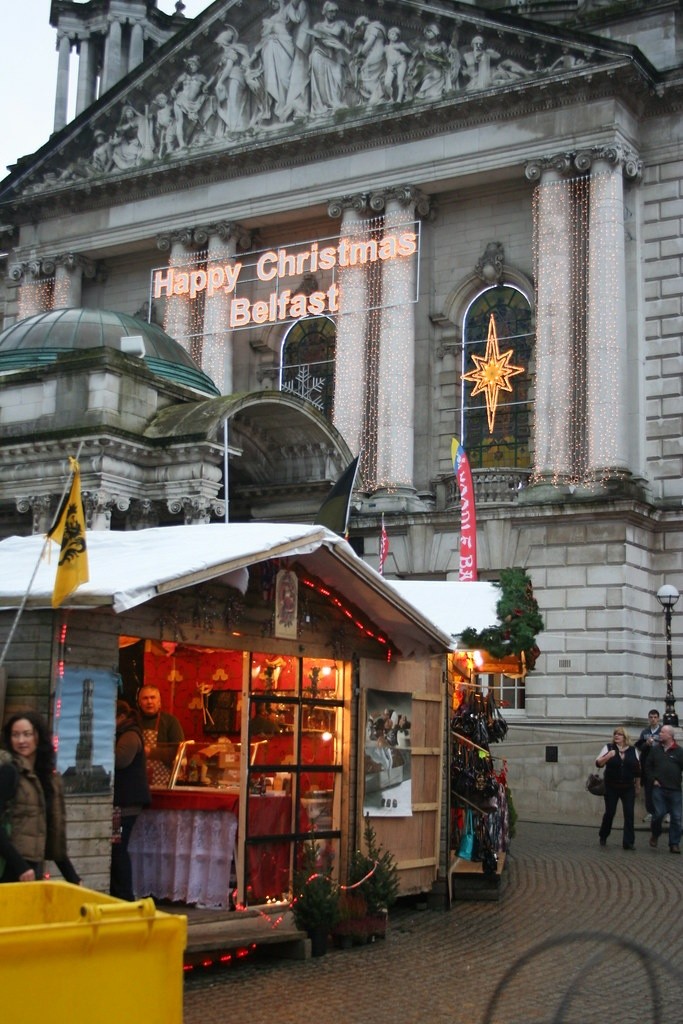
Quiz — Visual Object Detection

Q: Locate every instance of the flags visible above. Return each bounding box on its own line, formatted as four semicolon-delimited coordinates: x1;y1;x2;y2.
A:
43;457;89;609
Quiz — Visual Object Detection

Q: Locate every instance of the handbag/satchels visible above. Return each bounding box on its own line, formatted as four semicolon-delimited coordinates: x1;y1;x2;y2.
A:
585;774;607;796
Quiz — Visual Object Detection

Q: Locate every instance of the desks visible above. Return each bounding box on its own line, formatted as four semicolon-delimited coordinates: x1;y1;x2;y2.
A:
129;785;308;909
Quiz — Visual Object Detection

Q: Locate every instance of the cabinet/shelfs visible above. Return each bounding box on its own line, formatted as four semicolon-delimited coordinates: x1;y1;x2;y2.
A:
452;650;526;899
205;689;338;735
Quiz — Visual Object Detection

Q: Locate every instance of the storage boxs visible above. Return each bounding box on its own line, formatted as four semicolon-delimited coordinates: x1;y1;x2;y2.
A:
0;880;187;1024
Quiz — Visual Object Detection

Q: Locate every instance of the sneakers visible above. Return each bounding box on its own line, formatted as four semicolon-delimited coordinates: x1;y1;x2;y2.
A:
664;813;670;823
600;835;607;845
649;837;658;847
623;842;636;850
670;845;681;854
642;813;652;822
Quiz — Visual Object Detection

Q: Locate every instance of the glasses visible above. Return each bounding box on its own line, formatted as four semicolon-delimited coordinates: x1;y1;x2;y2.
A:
614;733;624;736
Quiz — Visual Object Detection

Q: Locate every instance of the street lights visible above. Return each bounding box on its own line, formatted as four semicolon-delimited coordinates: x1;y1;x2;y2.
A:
656;584;681;727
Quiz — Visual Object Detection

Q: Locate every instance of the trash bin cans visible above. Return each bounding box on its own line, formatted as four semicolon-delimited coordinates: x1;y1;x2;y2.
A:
0;878;186;1024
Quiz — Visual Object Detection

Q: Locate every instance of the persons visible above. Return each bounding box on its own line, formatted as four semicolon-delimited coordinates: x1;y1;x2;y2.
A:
644;725;683;854
24;1;588;195
595;726;641;851
634;708;672;823
111;698;153;907
131;685;186;787
0;709;85;887
